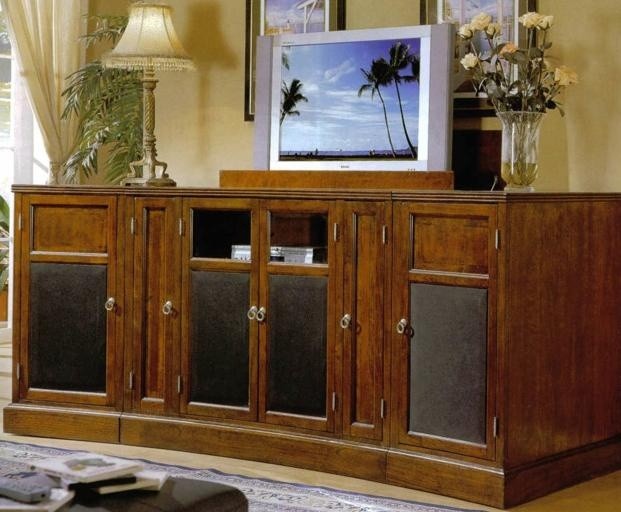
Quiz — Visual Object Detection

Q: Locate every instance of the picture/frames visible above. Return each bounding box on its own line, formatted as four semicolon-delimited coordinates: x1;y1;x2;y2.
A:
420;0;537;119
245;0;345;121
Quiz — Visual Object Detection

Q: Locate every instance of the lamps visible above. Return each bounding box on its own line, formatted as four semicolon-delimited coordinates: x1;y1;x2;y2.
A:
101;3;195;186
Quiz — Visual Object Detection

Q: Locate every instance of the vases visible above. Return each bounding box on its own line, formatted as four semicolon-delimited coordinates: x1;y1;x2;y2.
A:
496;112;544;192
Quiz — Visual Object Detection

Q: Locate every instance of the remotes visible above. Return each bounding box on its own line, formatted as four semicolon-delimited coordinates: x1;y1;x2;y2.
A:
0;475;52;503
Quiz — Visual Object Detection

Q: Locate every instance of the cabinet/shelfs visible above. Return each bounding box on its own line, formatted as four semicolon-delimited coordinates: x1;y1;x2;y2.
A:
1;184;126;446
128;185;393;481
386;192;620;510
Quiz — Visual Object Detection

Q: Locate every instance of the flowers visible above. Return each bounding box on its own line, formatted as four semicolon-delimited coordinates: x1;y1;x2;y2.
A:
457;10;580;164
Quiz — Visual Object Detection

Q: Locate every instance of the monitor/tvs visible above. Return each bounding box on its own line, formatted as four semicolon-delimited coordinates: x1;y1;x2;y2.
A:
254;24;453;171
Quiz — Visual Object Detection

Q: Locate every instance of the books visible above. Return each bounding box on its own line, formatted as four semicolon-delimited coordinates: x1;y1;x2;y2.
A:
133;464;172;492
25;450;143;482
47;471;138;491
87;476;160;493
1;486;77;511
